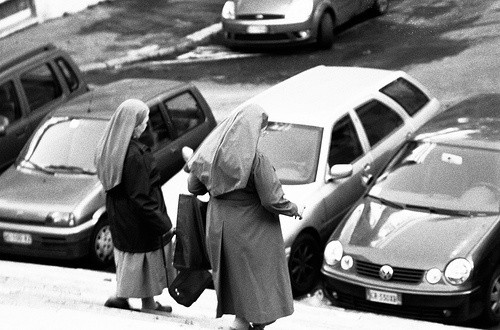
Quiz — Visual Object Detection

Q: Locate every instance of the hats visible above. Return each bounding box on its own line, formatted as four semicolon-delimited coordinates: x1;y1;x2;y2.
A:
189;103;269;197
93;99;149;191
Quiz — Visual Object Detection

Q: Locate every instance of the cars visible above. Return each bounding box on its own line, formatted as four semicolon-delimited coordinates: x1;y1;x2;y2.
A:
0;78;218;268
320;93;500;330
0;41;90;171
221;0;390;53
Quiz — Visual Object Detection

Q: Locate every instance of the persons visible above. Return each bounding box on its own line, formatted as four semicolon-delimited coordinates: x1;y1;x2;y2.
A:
93;99;173;315
190;105;303;330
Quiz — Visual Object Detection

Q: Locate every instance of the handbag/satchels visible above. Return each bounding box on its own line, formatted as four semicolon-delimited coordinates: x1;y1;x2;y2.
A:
169;269;214;307
174;193;212;271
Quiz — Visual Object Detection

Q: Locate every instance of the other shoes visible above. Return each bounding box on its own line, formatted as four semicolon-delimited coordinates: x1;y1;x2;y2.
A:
156;302;172;312
105;297;141;312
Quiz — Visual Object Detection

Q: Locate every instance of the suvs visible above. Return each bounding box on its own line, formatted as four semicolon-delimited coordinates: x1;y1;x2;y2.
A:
160;63;442;298
